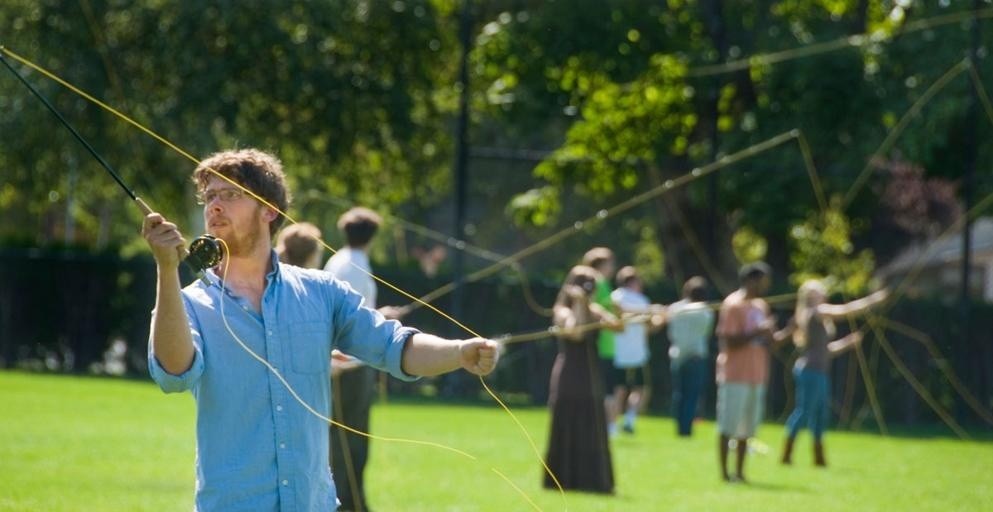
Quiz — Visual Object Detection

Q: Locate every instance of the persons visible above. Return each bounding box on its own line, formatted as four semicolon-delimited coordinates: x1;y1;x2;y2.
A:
715;263;784;483
782;281;888;464
653;273;715;433
584;245;653;434
271;221;401;376
543;263;615;495
143;148;499;512
322;206;382;509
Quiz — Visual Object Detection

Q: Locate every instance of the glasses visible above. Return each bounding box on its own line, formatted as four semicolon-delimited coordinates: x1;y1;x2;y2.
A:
201;186;253;201
